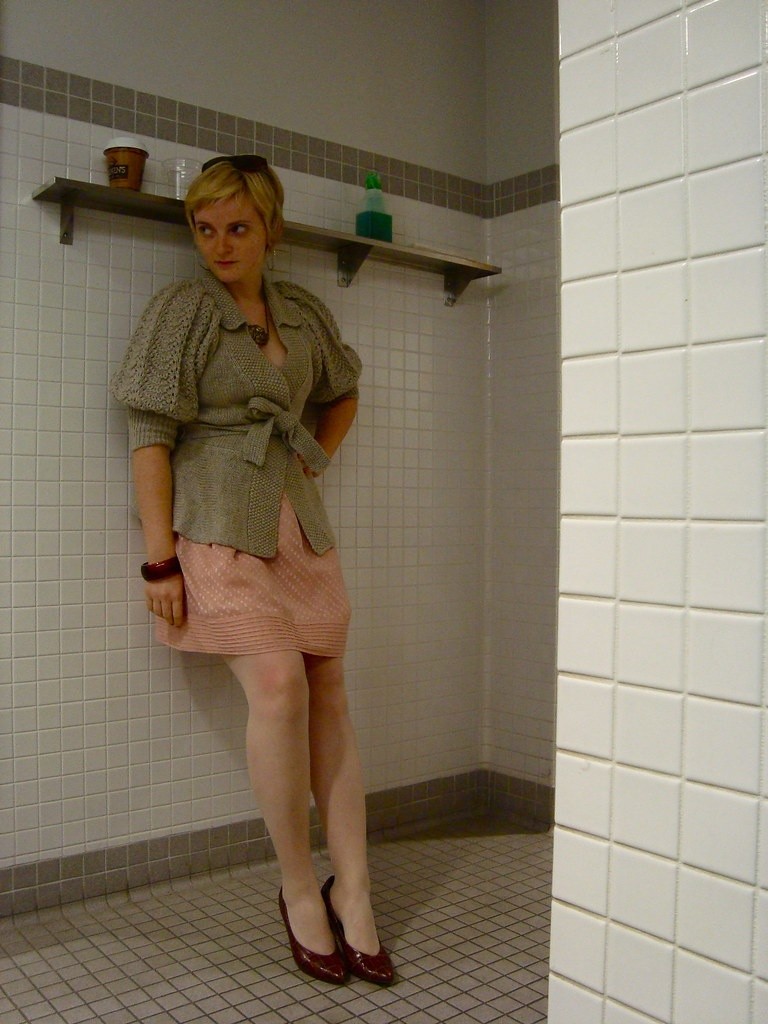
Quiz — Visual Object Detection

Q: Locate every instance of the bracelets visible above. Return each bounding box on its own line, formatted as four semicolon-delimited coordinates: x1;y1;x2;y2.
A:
141;554;181;582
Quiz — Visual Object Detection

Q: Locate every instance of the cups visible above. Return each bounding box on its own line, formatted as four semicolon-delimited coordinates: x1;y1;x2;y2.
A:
104;138;148;192
163;158;202;200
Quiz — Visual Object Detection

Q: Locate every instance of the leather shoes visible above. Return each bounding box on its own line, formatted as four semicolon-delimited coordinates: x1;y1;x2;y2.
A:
275;874;397;986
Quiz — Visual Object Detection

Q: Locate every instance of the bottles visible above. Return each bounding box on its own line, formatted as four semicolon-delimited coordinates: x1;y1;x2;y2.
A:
357;169;392;242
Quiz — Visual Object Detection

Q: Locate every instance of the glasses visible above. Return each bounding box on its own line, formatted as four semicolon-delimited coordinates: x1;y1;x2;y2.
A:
201;154;277;198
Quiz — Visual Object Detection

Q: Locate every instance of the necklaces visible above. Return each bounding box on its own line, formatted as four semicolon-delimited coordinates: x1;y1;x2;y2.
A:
244;299;272;348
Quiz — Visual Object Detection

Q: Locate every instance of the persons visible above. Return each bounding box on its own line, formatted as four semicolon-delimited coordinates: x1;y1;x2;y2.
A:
116;151;401;988
